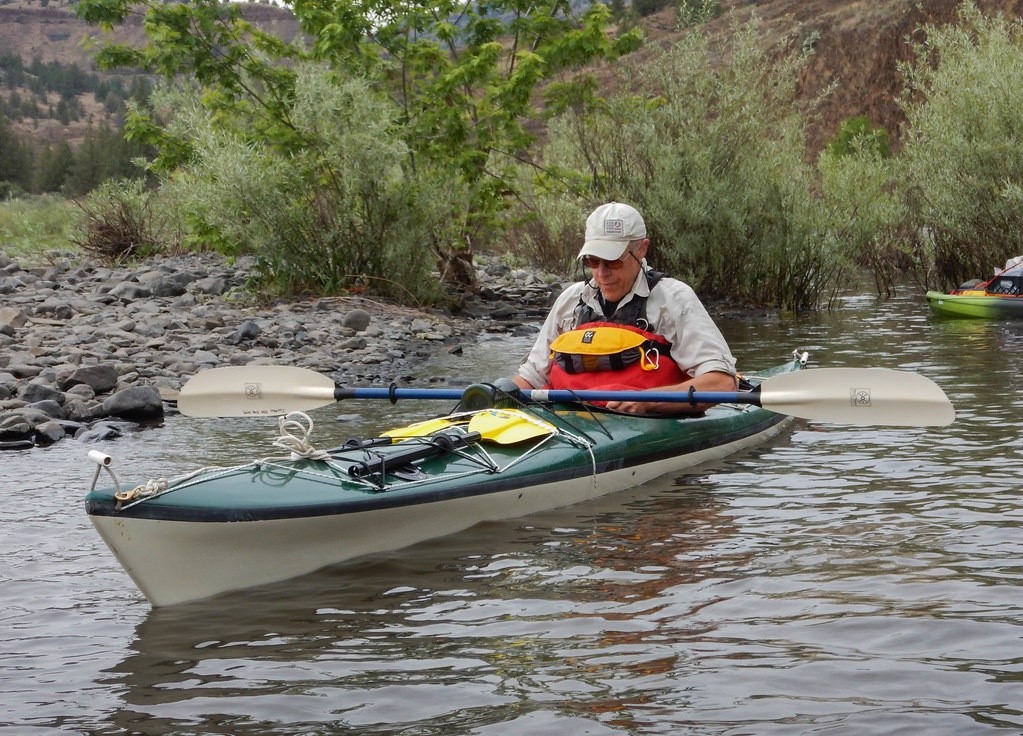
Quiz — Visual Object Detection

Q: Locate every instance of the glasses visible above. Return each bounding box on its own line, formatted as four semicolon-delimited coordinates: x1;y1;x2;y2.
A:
582;242;642;269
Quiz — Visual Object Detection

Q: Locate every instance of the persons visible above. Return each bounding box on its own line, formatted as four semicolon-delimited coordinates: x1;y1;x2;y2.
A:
513;201;739;415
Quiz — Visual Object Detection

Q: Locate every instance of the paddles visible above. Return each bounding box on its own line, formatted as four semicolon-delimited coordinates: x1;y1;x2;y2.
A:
323;418;454;453
347;408;557;478
175;364;957;431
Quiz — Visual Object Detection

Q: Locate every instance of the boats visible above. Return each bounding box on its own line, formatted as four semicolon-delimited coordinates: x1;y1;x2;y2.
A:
925;257;1023;320
79;348;805;608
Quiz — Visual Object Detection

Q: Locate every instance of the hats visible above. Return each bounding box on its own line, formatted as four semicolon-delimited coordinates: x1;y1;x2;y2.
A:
576;202;646;260
